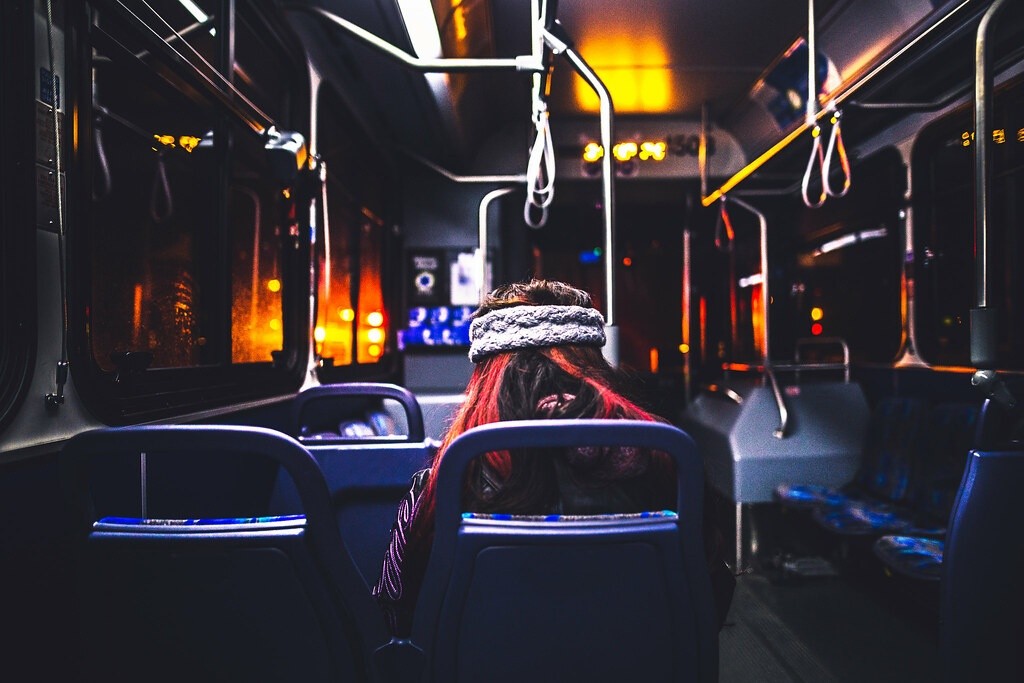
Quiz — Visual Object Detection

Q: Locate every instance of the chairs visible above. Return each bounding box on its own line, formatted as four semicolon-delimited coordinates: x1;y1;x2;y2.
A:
57;382;1023;683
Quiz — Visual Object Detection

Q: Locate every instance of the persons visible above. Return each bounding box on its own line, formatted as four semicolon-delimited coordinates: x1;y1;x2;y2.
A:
378;281;735;682
